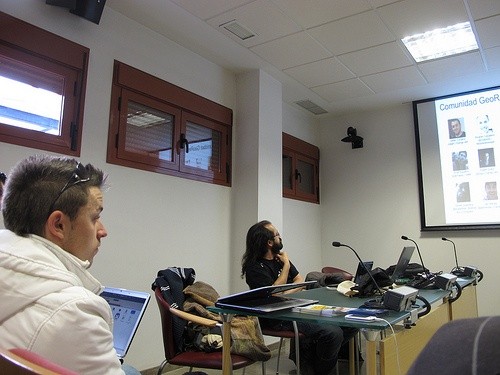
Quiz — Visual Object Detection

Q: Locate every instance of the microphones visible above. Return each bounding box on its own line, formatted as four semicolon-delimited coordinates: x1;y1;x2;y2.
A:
442;237;468;276
332;242;384;306
401;236;436;288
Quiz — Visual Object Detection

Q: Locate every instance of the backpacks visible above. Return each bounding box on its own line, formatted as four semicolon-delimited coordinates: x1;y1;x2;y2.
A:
305;272;345;290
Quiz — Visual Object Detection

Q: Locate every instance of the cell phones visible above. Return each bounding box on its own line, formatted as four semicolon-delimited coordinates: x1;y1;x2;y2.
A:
344;313;376;322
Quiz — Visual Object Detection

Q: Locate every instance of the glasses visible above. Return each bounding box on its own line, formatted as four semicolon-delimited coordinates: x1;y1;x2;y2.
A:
274;231;280;237
47;161;90;219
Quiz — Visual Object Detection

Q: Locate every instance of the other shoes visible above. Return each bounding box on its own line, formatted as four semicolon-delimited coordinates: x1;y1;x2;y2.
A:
290;351;304;369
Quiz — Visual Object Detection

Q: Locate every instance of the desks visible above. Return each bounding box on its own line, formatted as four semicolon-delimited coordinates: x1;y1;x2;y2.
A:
206;276;478;375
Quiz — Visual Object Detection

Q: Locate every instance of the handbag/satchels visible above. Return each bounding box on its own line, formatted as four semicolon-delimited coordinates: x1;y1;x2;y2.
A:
385;263;430;278
184;320;223;351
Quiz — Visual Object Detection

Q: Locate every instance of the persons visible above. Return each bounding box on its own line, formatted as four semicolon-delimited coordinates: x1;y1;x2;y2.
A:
477;115;493;134
241;221;361;375
450;119;465;138
452;151;468;171
478;148;495;168
0;152;127;375
457;182;470;202
485;182;498;200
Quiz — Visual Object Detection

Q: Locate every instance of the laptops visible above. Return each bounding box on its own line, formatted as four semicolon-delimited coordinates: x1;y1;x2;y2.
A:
388;245;415;281
329;262;373;288
215;280;320;314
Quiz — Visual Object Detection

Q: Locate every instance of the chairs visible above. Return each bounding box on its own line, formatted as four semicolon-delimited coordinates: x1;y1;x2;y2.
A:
154;270;266;375
262;328;304;375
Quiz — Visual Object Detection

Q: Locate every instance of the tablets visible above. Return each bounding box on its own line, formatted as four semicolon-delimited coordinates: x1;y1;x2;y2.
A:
98;286;151;363
